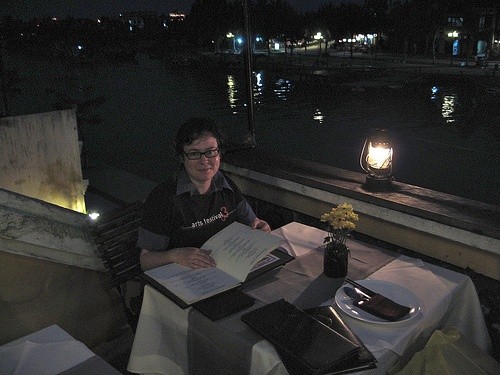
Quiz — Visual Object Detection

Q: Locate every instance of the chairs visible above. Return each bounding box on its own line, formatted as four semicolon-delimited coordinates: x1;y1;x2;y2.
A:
87;203;143;330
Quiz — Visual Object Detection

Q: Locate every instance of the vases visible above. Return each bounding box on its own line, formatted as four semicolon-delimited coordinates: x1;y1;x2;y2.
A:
323;242;348;279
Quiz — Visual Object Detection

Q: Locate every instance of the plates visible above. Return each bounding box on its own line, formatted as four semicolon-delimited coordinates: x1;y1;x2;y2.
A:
335;279;423;324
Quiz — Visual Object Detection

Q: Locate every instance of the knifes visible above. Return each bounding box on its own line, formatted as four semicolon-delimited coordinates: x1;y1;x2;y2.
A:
342;286;368;302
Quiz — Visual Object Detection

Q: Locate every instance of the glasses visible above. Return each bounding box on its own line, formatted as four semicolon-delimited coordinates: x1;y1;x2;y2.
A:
184;146;219;160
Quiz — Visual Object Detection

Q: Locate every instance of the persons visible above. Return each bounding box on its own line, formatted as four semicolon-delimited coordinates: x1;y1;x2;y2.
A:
137;116;271;271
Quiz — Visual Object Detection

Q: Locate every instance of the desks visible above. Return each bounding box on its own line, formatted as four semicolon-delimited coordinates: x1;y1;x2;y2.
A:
125;219;492;375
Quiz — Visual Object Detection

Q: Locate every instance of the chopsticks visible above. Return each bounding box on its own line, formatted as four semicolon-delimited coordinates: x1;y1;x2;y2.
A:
345;278;375;298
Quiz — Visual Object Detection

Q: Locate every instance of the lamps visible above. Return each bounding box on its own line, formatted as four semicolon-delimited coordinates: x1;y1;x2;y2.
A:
357;122;395;191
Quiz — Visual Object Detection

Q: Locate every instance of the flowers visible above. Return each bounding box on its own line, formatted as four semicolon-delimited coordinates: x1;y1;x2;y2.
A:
319;200;359;256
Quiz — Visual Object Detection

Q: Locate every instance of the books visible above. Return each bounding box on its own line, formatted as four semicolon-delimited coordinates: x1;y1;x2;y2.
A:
194;289;257;321
243;298;378;375
138;221;295;309
